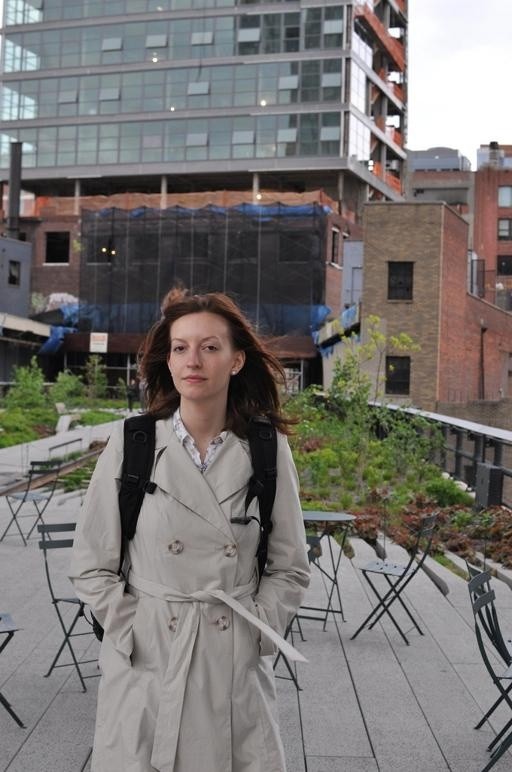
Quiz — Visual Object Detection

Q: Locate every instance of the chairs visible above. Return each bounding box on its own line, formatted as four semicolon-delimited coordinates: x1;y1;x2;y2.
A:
360;514;512;772
0;459;99;729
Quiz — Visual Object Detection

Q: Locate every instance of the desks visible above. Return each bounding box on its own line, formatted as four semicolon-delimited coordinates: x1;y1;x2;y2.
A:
298;510;356;632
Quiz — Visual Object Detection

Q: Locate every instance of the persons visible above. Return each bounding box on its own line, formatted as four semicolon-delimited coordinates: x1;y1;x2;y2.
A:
66;286;311;772
